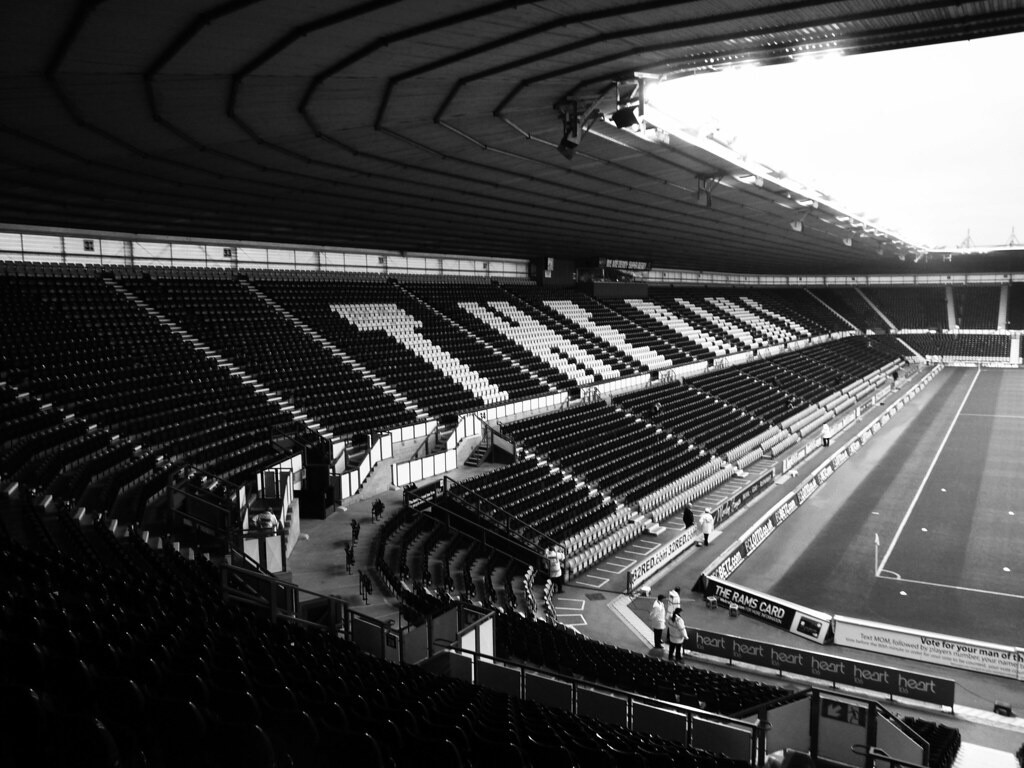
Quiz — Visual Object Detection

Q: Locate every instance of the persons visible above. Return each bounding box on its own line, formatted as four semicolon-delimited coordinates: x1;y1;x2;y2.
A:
650;586;689;662
820;422;831;447
258;507;279;529
699;508;714;547
786;396;801;410
345;543;356;575
405;482;417;501
893;370;898;381
351;518;360;546
683;502;695;529
654;402;663;415
542;545;565;593
372;498;385;521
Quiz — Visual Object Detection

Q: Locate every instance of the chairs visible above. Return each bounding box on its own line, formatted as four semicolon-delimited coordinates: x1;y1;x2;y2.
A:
0;261;1024;768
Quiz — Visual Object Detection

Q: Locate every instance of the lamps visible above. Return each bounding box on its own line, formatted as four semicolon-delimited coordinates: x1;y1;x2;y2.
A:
843;232;851;246
609;80;646;130
691;173;728;207
557;97;583;161
790;210;811;231
